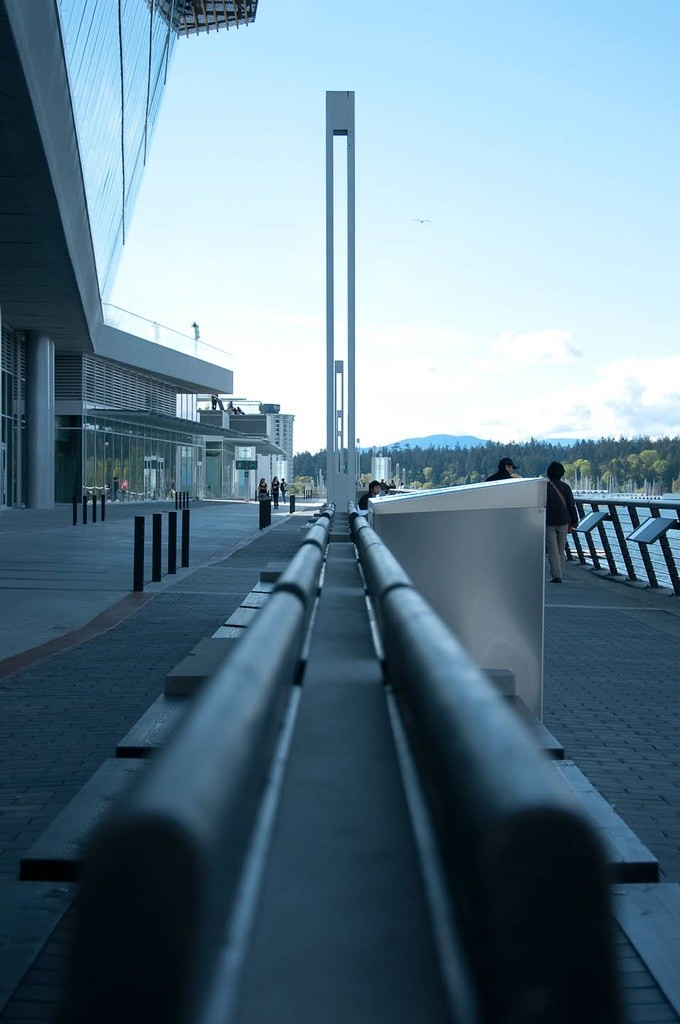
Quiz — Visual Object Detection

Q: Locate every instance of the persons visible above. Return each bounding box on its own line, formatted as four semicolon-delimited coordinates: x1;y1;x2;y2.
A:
483;457;518;482
110;477;128;503
545;461;579;583
258;478;268;500
271;476;280;509
280;478;288;504
357;478;404;511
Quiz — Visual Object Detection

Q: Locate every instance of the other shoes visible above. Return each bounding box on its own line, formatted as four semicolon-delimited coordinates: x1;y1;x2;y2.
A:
550;578;561;583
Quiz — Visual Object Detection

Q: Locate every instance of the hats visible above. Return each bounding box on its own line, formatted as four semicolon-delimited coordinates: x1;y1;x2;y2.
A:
500;458;518;469
